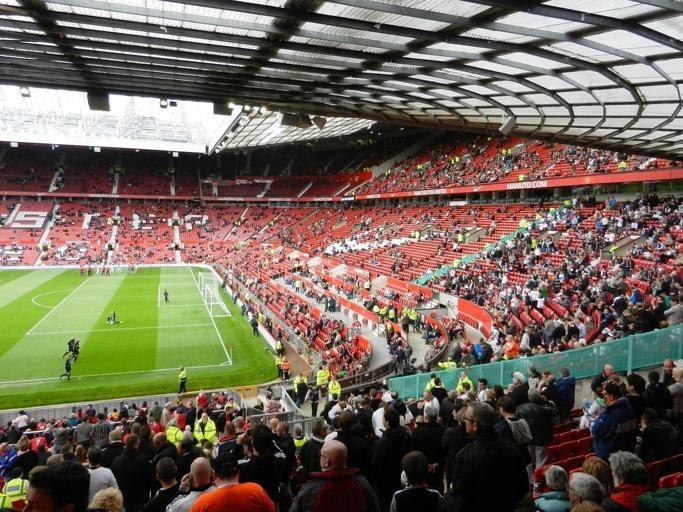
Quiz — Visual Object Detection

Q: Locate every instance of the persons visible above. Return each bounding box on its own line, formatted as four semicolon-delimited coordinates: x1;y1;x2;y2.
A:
1;337;682;511
216;127;683;336
106;310;122;325
0;155;214;275
163;288;170;305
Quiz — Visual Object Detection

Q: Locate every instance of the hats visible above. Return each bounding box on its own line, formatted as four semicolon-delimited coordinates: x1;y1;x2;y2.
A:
291;423;303;438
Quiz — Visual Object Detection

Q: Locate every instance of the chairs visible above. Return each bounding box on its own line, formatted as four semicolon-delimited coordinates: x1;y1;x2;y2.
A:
0;129;681;512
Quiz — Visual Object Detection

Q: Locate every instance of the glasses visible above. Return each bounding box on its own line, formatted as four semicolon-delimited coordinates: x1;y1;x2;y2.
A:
565;483;574;492
463;415;473;423
